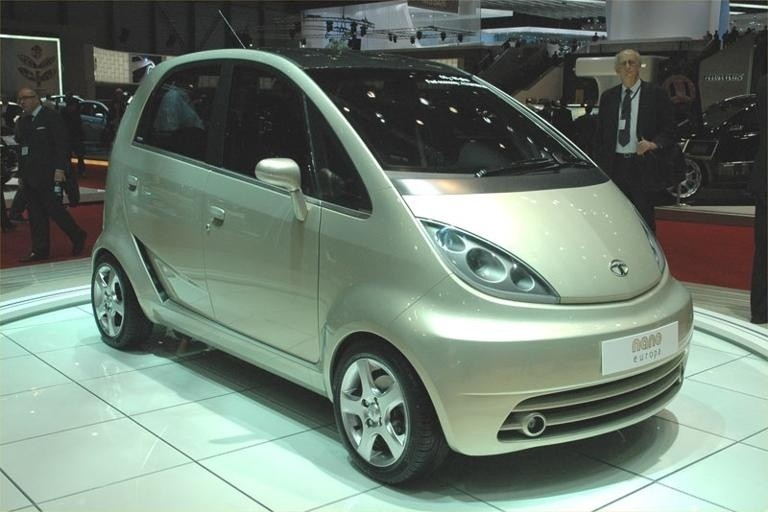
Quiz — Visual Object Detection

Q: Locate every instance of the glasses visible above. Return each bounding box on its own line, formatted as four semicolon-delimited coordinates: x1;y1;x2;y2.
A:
17;96;35;102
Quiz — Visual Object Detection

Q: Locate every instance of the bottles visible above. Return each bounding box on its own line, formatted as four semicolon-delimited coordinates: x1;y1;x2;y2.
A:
54;181;62;202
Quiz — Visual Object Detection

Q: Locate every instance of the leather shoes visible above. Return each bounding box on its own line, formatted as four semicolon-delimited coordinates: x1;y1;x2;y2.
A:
0;216;29;232
20;253;46;262
72;231;87;255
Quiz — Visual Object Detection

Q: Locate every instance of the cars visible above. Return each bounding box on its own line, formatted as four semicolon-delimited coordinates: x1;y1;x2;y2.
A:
664;93;760;203
0;95;112;186
91;48;693;484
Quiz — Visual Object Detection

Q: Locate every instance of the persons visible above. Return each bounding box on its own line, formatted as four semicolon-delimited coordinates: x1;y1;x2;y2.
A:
597;49;673;236
0;88;127;263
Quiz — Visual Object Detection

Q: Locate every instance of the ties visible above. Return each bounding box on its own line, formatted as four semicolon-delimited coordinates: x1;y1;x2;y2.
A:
618;89;632;147
